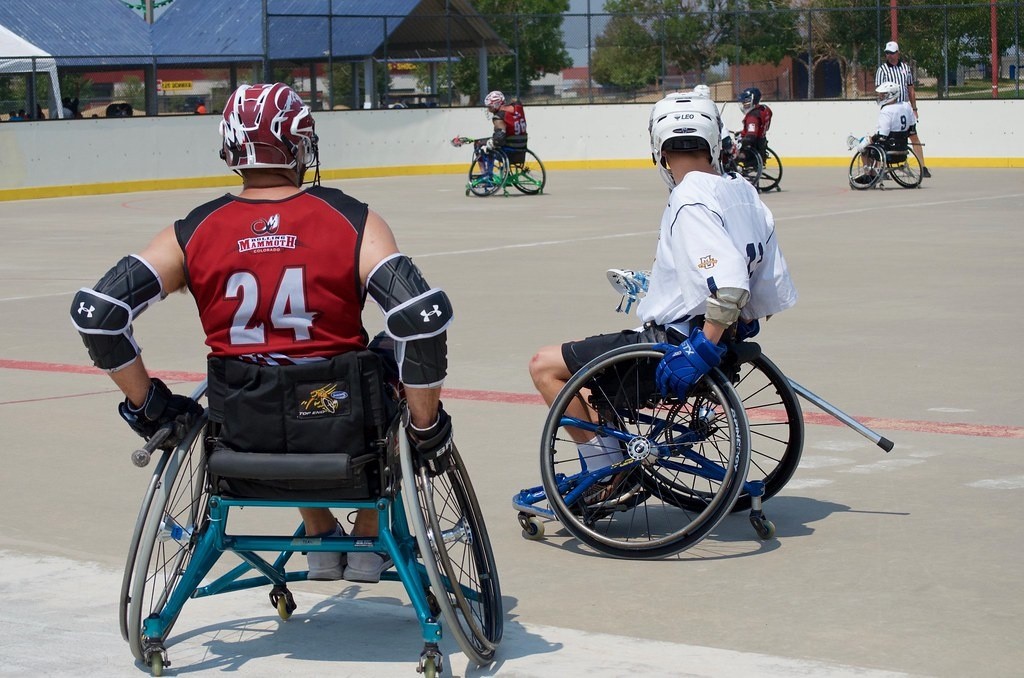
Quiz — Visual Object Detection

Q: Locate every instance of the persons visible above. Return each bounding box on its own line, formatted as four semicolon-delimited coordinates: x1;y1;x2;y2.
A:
874;41;931;178
62;96;80;119
853;81;911;185
474;90;528;187
721;87;773;175
694;85;711;98
194;98;206;114
67;82;453;584
9;103;45;121
527;91;794;512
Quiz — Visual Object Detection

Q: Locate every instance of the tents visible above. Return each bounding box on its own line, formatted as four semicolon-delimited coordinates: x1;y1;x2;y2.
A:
0;25;64;119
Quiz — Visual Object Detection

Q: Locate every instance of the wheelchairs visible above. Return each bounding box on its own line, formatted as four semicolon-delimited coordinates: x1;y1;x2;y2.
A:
848;129;924;190
464;139;548;197
513;314;805;561
117;352;506;678
718;135;783;194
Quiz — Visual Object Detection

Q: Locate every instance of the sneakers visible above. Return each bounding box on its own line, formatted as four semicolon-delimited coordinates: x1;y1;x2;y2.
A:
303;524;351;581
342;533;395;582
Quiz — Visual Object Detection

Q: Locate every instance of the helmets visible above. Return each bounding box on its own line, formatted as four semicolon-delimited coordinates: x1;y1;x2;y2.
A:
650;92;722;168
694;85;711;99
875;81;900;107
219;81;318;172
738;88;760;112
484;90;504;113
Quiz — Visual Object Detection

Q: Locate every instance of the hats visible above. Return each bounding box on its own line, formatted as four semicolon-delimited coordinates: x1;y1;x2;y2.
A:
883;41;900;53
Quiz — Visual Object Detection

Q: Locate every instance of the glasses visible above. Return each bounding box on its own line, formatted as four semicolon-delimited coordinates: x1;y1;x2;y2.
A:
885;51;898;55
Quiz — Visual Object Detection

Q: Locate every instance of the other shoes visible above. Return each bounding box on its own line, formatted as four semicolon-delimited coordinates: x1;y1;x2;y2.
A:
571;469;650;515
922;167;932;178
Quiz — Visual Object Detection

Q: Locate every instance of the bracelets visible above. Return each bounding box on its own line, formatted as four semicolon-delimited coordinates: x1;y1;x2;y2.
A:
913;108;918;111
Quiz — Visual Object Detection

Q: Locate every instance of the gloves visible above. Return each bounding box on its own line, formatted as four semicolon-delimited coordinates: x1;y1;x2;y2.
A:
654;324;729;406
402;403;452;479
116;375;206;449
